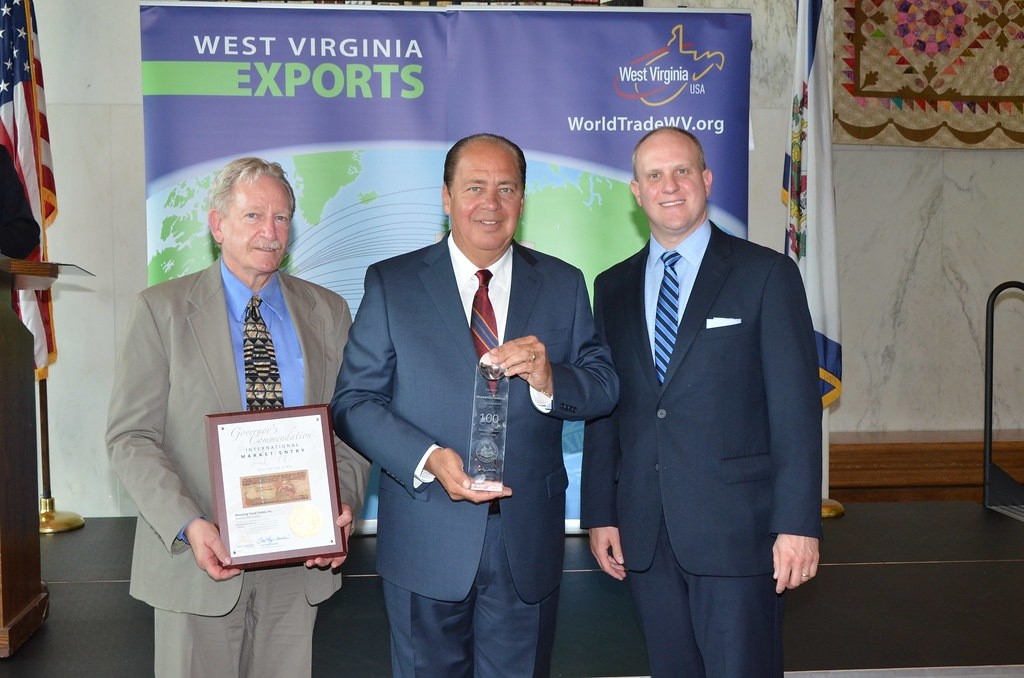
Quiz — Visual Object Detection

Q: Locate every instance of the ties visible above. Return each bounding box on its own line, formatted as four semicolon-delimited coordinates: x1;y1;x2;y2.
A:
655;251;682;385
244;295;283;412
470;270;499;395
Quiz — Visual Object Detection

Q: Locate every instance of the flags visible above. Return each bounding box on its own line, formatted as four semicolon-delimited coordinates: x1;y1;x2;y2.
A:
0;0;60;379
780;0;844;409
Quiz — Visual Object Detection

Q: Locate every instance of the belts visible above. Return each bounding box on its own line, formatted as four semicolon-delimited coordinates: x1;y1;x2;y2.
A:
489;502;501;515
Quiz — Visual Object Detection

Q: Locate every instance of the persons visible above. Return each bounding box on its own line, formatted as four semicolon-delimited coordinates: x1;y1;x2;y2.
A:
581;126;823;678
326;132;621;678
105;158;372;678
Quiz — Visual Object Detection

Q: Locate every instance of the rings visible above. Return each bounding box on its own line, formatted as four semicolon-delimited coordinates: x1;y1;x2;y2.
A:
801;573;810;577
532;353;536;361
